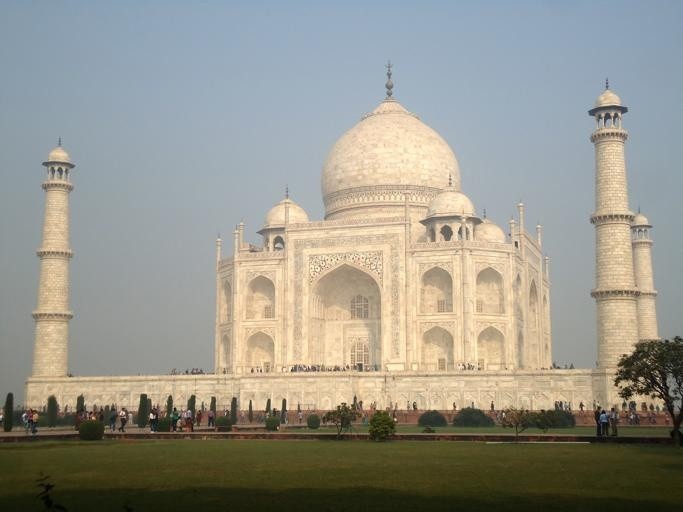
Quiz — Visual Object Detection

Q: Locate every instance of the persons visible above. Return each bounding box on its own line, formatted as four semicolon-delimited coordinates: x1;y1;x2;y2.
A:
349;400;417;411
592;405;670;439
554;401;570;411
454;360;481;371
471;402;474;408
578;402;584;411
535;360;574;369
168;364;380;375
452;402;455;411
501;411;506;421
20;406;229;433
490;401;494;410
